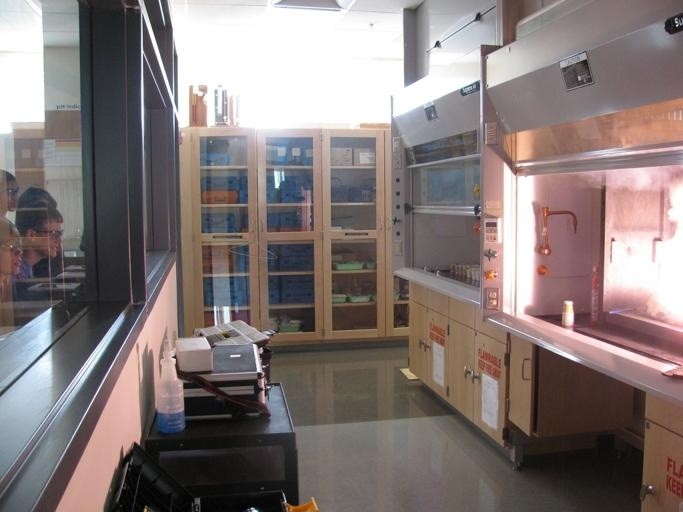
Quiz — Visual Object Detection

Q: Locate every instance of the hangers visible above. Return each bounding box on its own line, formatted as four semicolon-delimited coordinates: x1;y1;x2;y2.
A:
228;232;279;260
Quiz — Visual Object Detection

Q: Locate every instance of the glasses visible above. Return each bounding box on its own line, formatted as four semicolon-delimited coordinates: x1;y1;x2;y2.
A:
0;240;20;250
38;229;63;239
2;186;19;194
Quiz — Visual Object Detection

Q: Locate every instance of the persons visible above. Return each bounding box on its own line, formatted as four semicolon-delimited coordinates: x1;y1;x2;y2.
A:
0;169;65;328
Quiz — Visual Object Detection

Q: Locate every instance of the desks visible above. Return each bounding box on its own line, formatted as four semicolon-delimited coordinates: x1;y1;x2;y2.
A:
144;382;299;506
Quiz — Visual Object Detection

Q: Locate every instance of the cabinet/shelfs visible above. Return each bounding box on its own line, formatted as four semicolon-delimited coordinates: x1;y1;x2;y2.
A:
639;392;683;512
448;297;508;448
322;129;409;341
408;281;448;402
508;333;634;439
179;128;323;347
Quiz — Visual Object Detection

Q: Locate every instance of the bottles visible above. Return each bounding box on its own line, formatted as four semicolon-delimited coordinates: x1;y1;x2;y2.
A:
561;300;575;326
213;83;229;127
155;340;186;435
